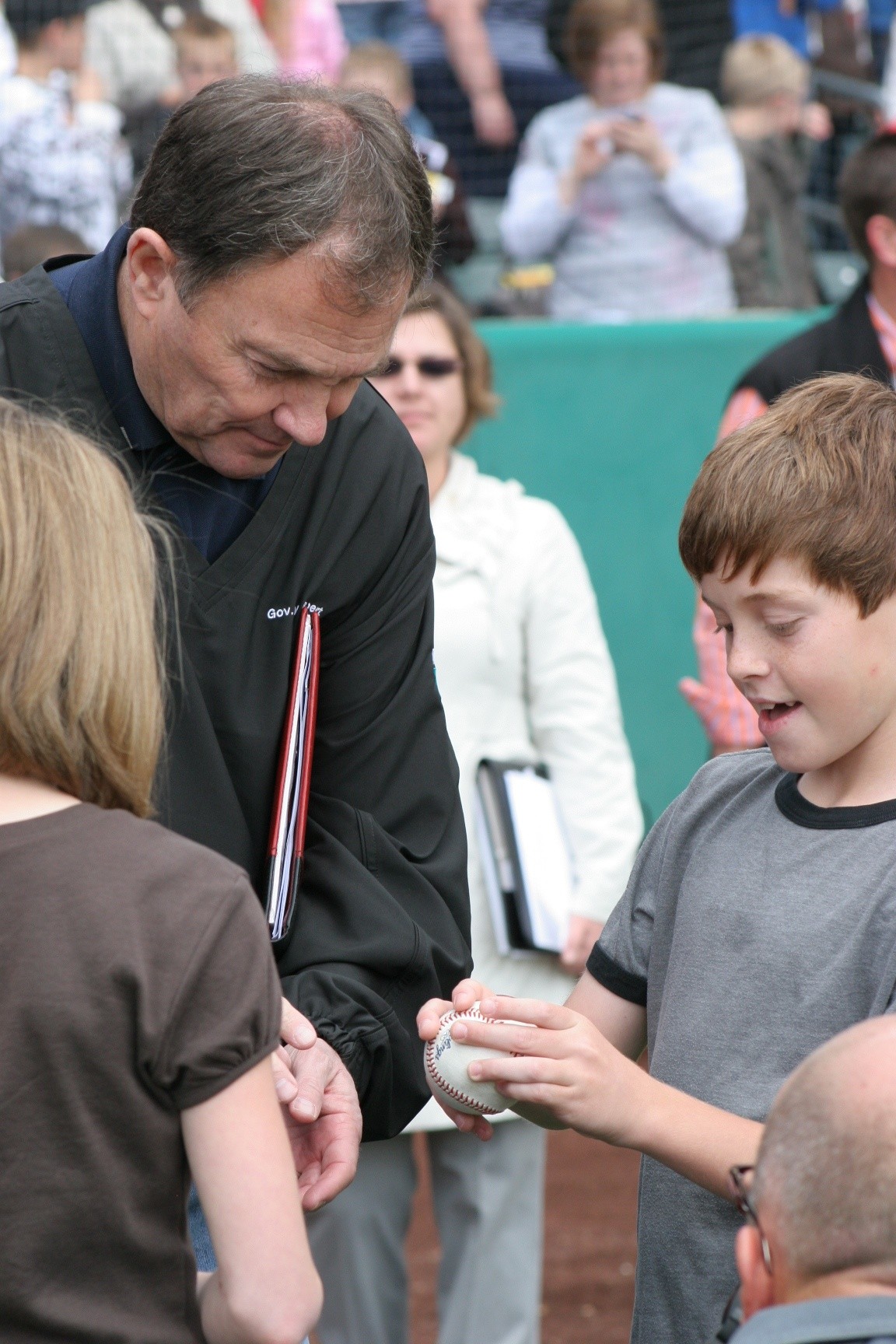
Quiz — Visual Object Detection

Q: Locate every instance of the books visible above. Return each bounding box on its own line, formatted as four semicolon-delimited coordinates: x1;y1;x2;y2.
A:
263;607;319;941
471;758;582;962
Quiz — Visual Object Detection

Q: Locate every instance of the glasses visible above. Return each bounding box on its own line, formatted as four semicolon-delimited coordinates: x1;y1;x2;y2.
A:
383;357;458;375
730;1166;773;1272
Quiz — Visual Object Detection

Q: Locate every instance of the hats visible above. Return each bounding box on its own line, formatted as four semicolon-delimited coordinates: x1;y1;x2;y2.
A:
5;0;109;16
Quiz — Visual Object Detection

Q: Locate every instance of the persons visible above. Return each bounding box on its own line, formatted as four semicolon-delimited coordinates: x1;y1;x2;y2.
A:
0;0;896;293
680;128;896;761
496;0;749;314
421;365;896;1344
0;397;323;1344
732;1010;896;1344
304;280;650;1344
718;33;829;311
0;76;473;1209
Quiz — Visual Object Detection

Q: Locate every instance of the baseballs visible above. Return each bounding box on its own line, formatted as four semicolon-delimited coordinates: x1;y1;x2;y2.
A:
423;999;538;1116
